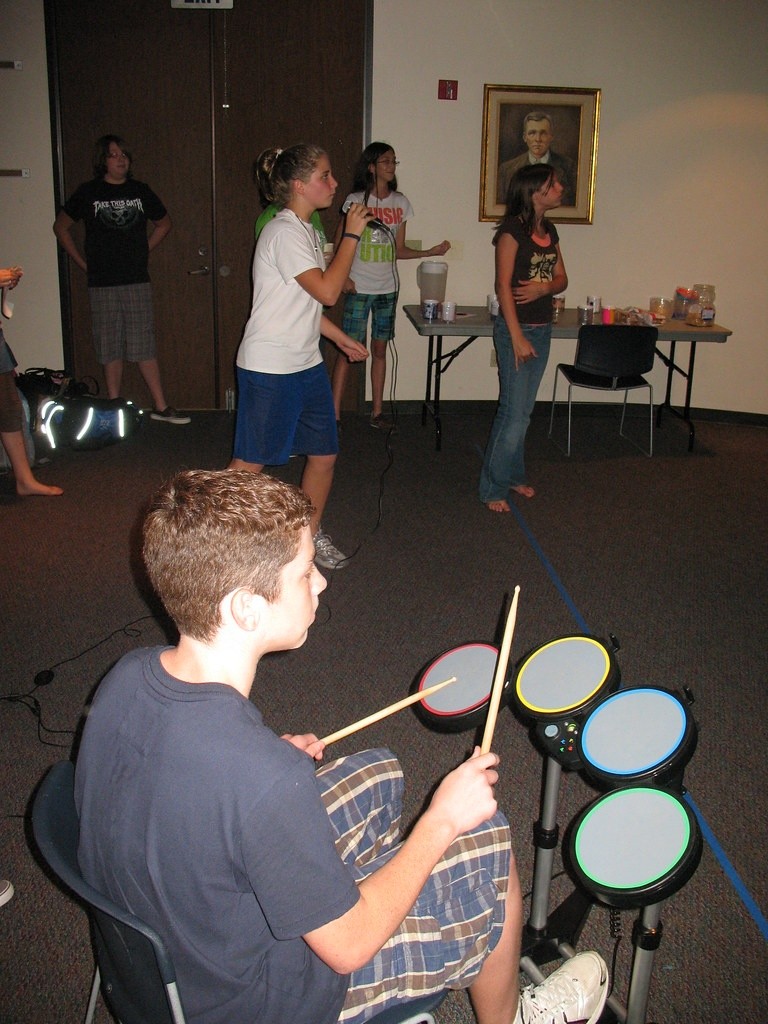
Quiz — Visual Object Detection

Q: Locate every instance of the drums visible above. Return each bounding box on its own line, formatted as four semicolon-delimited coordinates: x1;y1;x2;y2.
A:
561;787;702;908
407;641;511;735
578;685;696;788
513;632;622;730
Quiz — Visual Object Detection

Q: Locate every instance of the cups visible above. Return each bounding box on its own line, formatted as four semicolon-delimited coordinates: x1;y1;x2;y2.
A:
552;294;565;314
577;305;593;324
491;301;499;316
487;294;497;311
586;295;601;313
423;299;438;320
441;301;456;322
601;306;615;325
650;296;674;321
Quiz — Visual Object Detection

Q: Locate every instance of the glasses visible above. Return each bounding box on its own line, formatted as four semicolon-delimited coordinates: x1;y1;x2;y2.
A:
377;157;400;166
107;152;128;159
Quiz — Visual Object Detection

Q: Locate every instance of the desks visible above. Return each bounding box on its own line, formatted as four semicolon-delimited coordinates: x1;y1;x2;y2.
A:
403;305;732;452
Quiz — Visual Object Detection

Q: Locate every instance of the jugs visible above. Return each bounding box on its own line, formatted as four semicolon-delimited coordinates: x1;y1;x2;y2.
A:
416;261;448;311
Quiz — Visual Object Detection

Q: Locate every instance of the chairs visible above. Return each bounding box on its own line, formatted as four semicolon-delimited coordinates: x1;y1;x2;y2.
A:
32;761;449;1024
548;324;658;458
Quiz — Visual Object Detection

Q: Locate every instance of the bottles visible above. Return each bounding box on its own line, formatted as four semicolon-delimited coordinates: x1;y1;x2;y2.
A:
674;283;717;327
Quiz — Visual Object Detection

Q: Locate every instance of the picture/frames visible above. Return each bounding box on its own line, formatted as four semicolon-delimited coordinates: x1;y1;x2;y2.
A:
478;84;602;225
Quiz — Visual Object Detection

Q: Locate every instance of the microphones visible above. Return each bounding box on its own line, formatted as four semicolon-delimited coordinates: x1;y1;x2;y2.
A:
341;200;390;232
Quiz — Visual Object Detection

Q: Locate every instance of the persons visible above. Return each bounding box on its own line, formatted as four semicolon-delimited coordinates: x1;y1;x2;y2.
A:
254;207;358;311
332;143;450;434
53;135;191;422
479;165;568;512
73;471;609;1024
225;139;375;568
0;267;63;495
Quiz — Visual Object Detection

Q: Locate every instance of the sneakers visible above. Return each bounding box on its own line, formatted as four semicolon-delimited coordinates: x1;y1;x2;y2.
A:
312;525;349;569
513;951;609;1024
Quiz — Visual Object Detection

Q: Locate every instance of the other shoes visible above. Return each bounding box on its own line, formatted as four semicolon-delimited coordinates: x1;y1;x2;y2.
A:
336;421;344;442
368;413;398;431
150;402;191;425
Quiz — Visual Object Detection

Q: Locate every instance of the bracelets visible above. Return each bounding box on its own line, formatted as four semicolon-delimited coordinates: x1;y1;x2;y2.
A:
343;233;360;241
